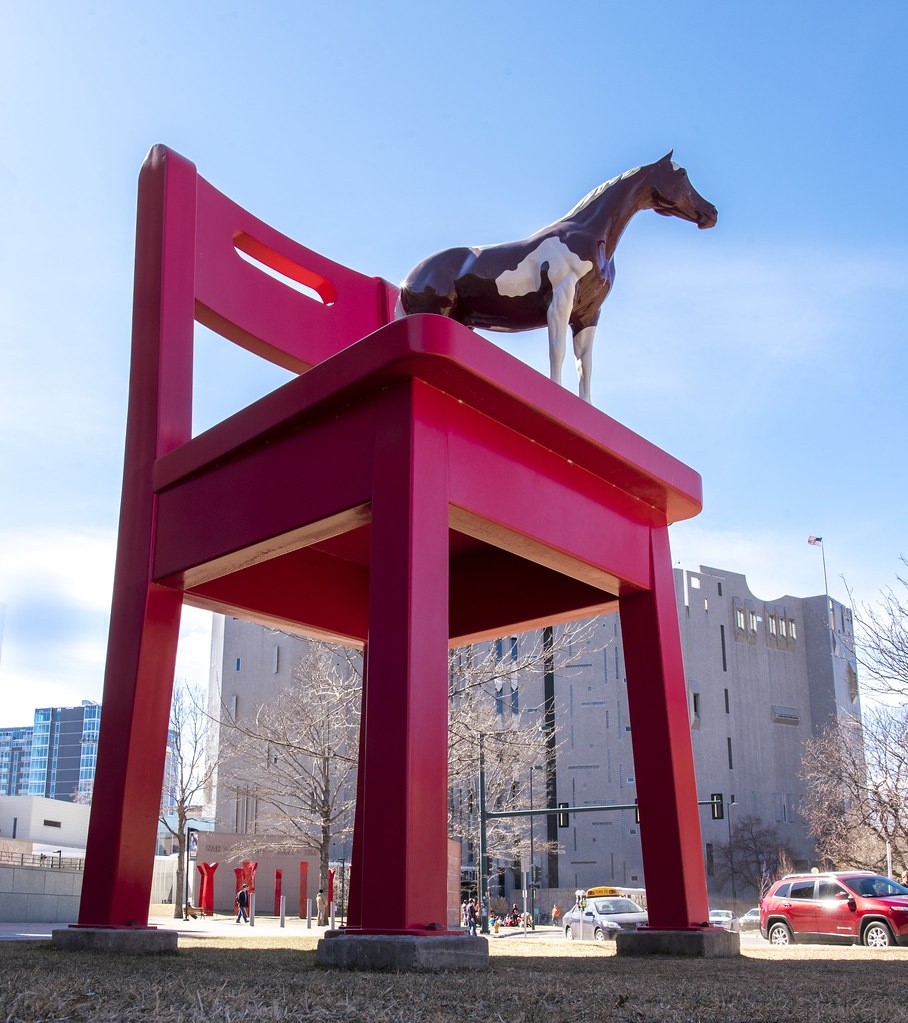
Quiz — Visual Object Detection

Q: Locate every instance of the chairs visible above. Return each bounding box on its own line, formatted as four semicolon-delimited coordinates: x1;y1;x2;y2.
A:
68;141;725;934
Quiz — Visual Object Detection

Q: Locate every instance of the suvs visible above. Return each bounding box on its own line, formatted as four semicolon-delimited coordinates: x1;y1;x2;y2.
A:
760;866;908;947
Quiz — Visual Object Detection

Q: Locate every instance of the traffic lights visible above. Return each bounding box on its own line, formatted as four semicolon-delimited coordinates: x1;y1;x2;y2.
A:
534;865;542;883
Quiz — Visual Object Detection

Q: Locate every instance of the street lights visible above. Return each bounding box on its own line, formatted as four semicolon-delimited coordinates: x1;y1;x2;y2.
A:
338;858;345;927
529;766;543;930
727;802;737;908
183;827;199;920
53;850;62;869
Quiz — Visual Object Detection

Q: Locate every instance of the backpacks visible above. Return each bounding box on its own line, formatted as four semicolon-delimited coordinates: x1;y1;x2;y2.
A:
514;910;519;915
316;896;323;907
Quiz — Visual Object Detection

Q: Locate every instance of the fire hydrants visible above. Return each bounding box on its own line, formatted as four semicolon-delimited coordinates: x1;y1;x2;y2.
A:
493;920;500;932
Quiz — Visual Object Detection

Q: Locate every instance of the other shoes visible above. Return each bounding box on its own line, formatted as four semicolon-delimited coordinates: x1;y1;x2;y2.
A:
245;920;249;923
236;921;241;923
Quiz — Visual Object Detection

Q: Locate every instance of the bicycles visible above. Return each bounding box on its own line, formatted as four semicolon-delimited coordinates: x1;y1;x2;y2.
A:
539;911;553;925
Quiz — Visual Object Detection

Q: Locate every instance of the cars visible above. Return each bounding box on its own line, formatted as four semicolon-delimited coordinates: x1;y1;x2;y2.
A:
739;907;762;932
562;886;649;942
709;910;741;934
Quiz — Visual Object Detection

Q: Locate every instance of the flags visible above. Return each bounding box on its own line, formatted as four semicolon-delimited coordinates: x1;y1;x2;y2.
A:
808;536;822;547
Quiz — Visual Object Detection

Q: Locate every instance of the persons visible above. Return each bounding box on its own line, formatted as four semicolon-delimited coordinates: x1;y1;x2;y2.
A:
190;834;197;851
551;905;560;926
236;884;249;923
462;897;531;936
899;870;908;888
187;901;198;919
317;889;327;926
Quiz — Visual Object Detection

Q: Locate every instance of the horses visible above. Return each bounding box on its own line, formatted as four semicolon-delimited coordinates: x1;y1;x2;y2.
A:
400;149;717;403
